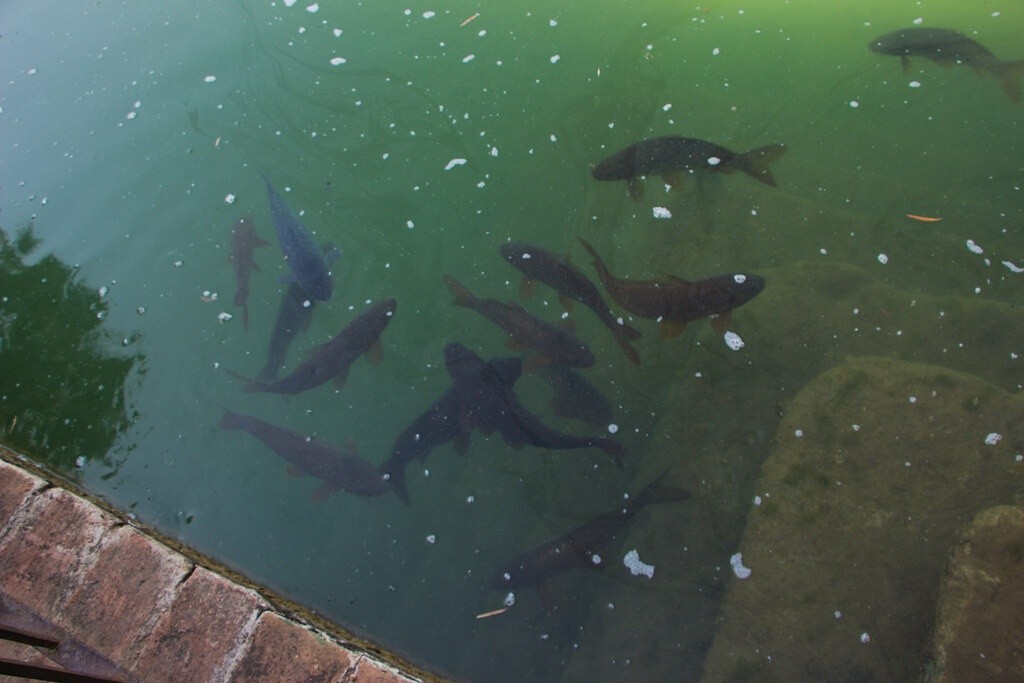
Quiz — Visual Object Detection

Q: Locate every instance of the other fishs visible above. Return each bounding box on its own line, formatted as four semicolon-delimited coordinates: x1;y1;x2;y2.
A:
216;170;767;591
869;27;1024;104
590;133;788;188
